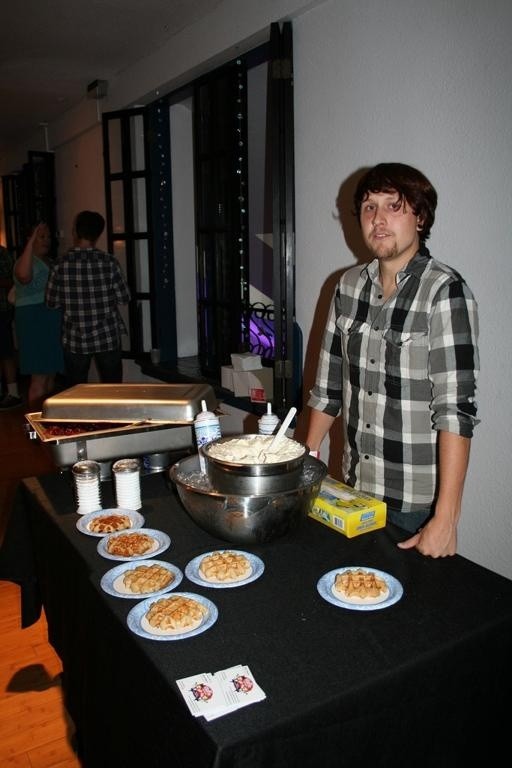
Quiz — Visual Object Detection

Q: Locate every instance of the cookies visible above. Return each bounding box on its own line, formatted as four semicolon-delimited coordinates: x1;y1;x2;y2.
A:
107;532;154;557
145;595;208;631
198;551;251;581
90;514;131;533
123;564;175;595
332;568;390;604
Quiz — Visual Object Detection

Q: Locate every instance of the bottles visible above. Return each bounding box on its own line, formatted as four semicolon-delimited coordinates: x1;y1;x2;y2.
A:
72;460;105;513
109;460;144;511
257;401;281;436
193;399;224;476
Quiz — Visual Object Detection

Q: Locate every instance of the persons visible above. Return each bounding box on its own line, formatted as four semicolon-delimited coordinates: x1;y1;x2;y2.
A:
294;159;482;559
44;210;132;383
12;218;65;407
1;241;24;414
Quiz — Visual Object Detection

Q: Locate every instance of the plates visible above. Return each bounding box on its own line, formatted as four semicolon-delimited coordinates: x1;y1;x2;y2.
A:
317;567;402;613
96;526;173;560
131;593;219;639
75;507;147;536
185;548;266;588
101;559;185;599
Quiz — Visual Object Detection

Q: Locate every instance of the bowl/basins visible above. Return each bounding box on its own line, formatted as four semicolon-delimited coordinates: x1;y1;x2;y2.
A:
169;452;329;543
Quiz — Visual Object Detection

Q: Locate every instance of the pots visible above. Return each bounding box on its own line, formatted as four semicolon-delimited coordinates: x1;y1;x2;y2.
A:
196;433;311;495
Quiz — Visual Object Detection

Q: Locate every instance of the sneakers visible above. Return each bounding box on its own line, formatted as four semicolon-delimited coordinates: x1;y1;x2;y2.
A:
0;393;25;411
